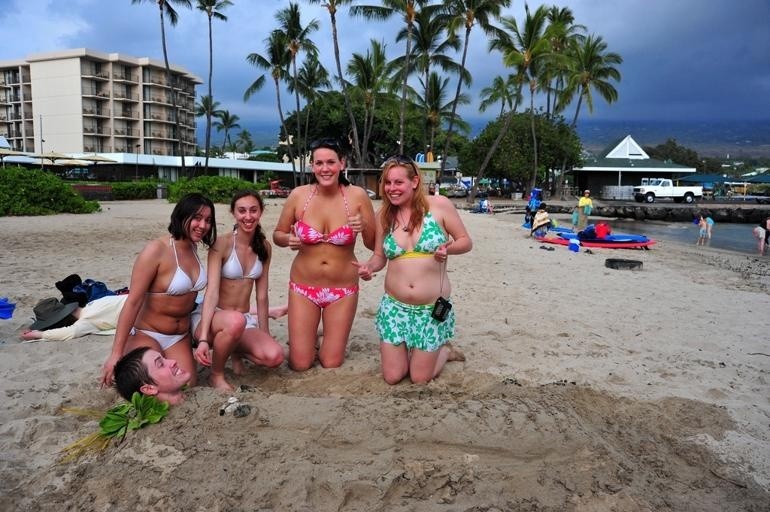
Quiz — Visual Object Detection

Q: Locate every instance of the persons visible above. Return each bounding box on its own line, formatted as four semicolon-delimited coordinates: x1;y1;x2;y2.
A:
579;187;593;230
754;217;770;254
698;215;706;244
347;154;467;385
530;203;552;239
191;191;285;392
111;345;190;407
270;136;377;372
100;192;218;390
20;297;294;343
705;213;714;239
572;206;580;226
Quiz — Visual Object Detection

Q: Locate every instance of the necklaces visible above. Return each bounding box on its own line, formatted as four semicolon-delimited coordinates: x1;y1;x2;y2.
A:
395;207;412;233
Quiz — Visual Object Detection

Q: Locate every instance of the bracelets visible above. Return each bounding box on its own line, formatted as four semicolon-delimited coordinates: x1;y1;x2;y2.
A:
197;338;209;343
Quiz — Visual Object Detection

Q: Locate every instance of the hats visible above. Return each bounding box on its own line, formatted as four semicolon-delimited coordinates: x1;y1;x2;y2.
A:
29;296;79;331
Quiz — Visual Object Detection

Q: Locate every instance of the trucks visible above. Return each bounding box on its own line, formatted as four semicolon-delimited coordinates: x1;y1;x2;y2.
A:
633;177;704;203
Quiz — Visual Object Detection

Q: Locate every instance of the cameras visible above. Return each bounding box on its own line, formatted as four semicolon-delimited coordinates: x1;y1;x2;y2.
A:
431;297;453;323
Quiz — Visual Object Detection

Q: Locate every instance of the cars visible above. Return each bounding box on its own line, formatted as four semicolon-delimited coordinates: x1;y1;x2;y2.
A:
756;189;770;205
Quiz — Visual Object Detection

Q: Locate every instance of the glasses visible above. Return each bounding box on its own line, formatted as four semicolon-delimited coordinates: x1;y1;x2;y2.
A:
308;137;341;150
385;154;418;176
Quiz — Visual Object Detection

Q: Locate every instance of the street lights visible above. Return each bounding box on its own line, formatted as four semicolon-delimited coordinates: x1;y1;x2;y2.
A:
40;137;45;170
135;142;141;181
